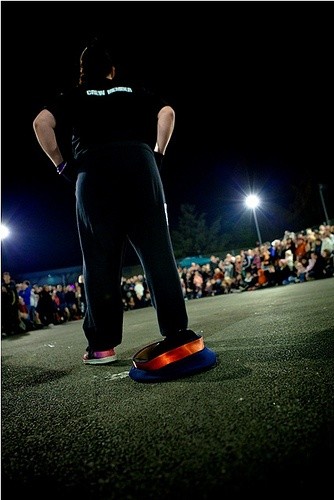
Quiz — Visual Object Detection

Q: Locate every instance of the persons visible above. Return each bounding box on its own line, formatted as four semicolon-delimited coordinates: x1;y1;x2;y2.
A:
32;44;189;364
1;225;334;341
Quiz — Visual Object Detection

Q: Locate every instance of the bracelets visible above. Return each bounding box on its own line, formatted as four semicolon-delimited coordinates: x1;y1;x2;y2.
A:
56;160;67;174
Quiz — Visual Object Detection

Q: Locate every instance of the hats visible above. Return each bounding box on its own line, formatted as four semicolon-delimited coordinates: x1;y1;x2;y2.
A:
128;329;217;381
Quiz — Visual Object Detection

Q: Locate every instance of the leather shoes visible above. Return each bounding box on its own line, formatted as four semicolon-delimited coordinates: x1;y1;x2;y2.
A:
82;346;118;364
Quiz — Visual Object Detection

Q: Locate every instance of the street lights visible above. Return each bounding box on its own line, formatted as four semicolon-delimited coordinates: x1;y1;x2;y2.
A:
245;194;262;245
318;183;331;225
